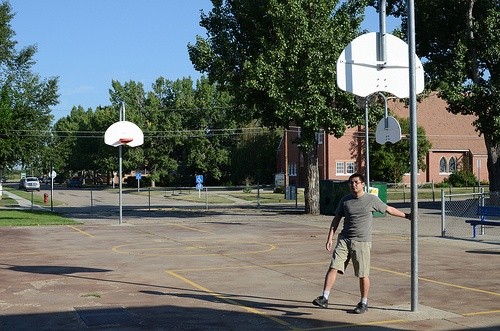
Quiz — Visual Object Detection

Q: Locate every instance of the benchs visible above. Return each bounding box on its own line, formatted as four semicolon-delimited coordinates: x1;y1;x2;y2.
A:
465;206;500;237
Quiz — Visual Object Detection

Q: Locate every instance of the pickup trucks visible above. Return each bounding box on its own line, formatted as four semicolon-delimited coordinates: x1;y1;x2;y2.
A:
85;175;104;185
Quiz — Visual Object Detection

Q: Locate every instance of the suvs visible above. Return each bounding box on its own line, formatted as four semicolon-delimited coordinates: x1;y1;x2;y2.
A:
18;175;42;192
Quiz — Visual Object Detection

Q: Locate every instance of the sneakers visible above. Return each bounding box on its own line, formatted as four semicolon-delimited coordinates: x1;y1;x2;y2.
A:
313;296;328;308
354;302;368;313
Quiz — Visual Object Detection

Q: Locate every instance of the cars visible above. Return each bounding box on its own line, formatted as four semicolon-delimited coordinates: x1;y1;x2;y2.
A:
68;177;82;189
38;178;43;183
44;177;55;185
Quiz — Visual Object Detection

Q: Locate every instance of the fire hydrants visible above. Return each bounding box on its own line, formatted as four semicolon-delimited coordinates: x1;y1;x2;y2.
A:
44;193;49;205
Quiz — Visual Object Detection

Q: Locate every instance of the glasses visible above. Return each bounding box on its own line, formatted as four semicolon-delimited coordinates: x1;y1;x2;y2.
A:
348;182;363;185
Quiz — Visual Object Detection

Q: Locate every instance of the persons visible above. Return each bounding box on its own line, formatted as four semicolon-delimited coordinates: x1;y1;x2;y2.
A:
313;174;411;313
